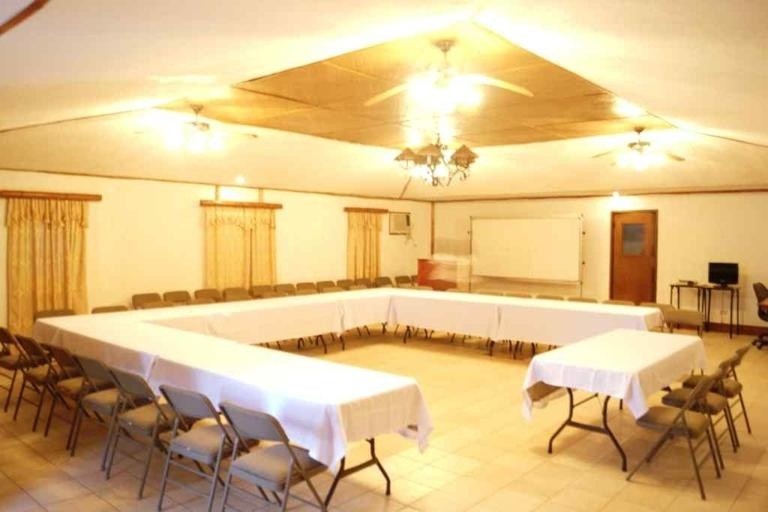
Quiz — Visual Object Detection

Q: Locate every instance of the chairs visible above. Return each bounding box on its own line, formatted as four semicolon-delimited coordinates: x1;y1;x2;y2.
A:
752;282;768;349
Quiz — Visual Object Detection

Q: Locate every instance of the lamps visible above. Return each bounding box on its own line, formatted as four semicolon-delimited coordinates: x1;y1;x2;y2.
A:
394;134;478;187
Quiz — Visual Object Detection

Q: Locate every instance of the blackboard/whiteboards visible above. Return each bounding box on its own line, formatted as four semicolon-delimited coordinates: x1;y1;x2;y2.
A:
469;216;581;283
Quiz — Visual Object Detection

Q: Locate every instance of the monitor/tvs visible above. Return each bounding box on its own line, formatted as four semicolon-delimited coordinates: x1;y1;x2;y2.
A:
708;262;739;290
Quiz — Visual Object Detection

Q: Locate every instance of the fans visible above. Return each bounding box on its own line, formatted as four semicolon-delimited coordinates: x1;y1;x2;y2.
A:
364;38;534;107
591;127;685;162
